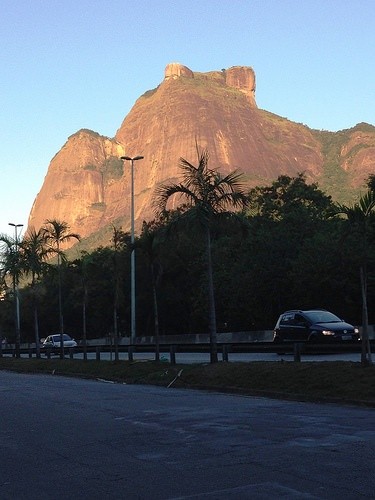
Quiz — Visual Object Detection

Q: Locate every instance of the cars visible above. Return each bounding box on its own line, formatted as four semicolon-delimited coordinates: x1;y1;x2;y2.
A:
43;334;77;356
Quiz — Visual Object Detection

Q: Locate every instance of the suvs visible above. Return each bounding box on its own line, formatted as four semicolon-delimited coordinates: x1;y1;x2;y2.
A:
274;309;360;356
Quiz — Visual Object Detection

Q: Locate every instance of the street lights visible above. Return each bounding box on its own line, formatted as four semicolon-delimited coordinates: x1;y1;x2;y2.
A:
9;223;23;358
121;156;146;363
